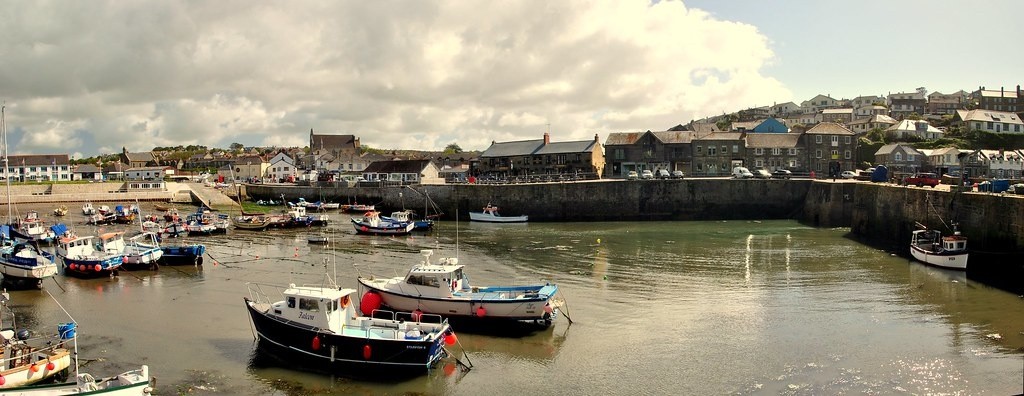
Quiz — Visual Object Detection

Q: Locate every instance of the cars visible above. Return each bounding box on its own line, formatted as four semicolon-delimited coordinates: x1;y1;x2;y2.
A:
656;169;670;180
627;171;638;180
670;170;684;180
840;171;858;179
641;170;653;179
754;169;773;180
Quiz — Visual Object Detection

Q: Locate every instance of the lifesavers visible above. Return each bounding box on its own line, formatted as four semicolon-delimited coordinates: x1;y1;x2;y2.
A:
341;295;350;308
197;257;203;266
452;279;458;292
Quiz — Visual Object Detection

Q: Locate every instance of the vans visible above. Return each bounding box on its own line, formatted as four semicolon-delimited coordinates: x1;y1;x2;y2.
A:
733;167;754;180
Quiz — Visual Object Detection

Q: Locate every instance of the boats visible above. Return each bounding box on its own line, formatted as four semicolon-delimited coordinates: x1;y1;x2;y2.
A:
354;207;558;329
909;192;969;270
0;102;445;283
0;330;153;396
0;288;79;389
242;219;451;379
469;201;529;223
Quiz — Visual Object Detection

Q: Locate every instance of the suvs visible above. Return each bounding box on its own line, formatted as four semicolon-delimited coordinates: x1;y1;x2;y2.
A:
903;173;939;188
771;170;791;180
